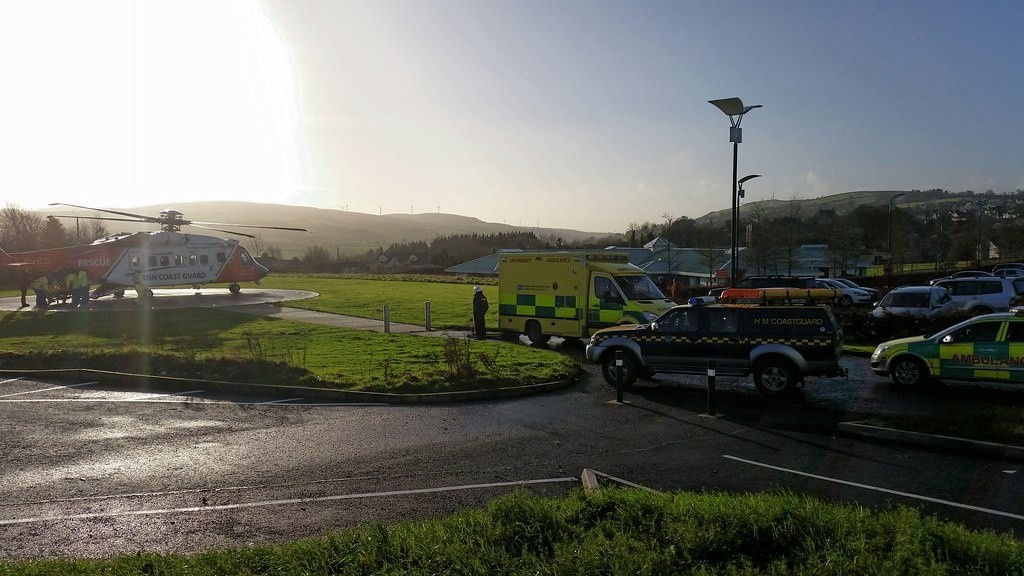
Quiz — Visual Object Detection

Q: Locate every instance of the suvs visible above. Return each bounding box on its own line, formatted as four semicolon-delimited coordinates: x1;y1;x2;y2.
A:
585;296;849;398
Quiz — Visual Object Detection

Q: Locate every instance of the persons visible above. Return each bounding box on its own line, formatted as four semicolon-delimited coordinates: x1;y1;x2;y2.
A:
656;276;665;289
65;266;89;307
473;286;489;341
32;272;54;306
16;267;30;308
667;278;679;298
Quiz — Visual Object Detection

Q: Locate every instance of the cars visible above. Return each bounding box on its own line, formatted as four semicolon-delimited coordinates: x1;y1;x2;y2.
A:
816;278;872;306
932;276;1016;318
930;263;1024;304
833;278;878;299
869;285;957;318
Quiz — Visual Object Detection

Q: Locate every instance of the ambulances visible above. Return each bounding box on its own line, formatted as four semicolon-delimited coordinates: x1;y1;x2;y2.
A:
497;249;679;344
870;307;1024;391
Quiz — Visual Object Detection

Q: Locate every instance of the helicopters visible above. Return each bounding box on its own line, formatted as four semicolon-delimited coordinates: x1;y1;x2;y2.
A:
0;202;309;300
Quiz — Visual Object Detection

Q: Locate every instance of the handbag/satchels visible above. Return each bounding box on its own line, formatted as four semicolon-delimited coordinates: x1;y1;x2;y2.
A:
675;287;687;298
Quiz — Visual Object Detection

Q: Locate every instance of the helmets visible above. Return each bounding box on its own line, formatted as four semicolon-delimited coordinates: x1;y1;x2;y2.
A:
658;276;664;280
473;285;483;292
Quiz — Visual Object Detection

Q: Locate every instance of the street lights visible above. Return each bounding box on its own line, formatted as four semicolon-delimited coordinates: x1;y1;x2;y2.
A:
709;96;762;290
